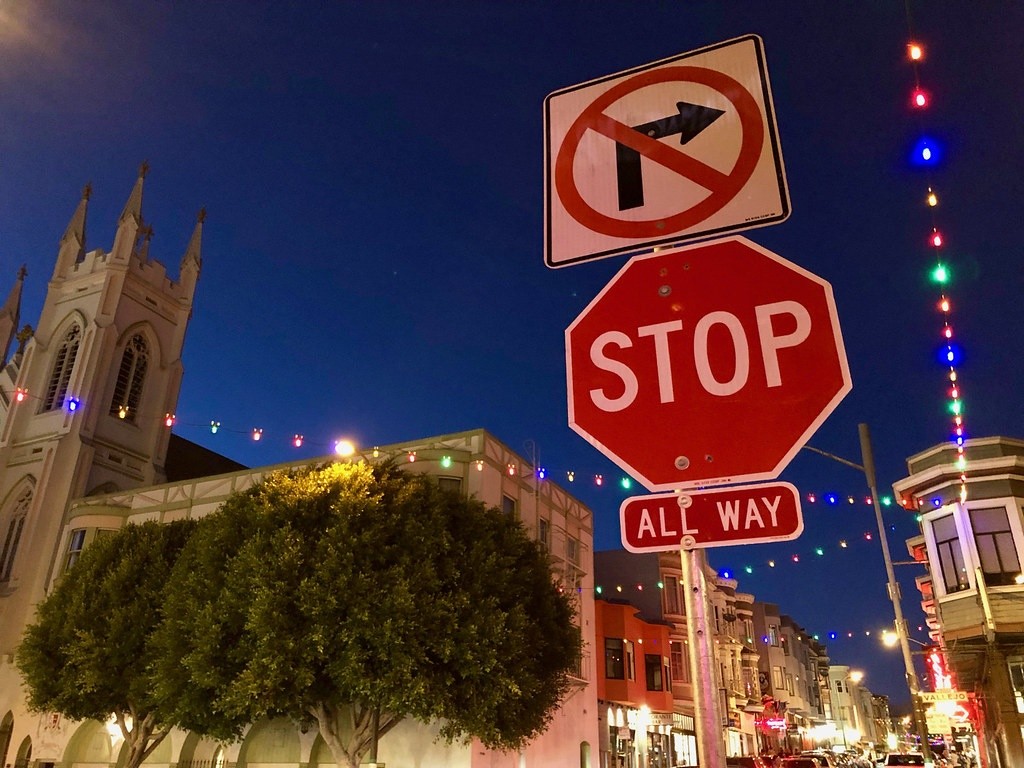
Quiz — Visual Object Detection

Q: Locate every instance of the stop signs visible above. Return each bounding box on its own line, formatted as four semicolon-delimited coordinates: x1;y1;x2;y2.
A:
566;234;854;492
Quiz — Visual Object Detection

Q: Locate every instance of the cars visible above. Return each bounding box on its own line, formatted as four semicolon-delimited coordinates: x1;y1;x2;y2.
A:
726;748;926;768
